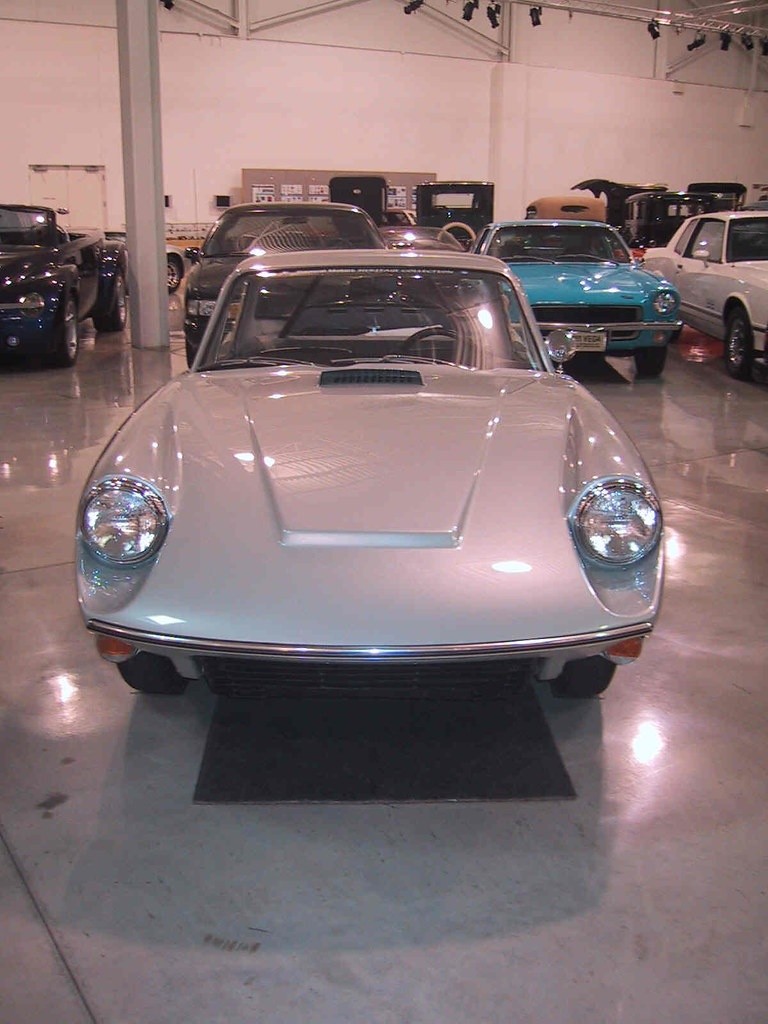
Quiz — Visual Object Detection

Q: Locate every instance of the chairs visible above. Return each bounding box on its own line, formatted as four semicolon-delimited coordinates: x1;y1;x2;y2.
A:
497;241;530;256
567;240;600;257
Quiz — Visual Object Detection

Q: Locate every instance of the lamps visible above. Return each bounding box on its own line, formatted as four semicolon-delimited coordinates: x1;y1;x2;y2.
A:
403;0;423;14
687;29;706;51
462;0;478;21
486;0;501;28
647;18;660;40
527;7;543;27
741;33;755;50
719;30;732;50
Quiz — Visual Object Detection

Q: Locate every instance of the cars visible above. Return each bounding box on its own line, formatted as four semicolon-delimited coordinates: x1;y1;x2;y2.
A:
106;234;189;296
181;201;404;371
376;226;490;357
468;220;683;378
78;246;666;699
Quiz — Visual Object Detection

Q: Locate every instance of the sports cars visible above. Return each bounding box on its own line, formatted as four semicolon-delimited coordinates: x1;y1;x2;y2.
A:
643;210;768;382
0;203;127;368
